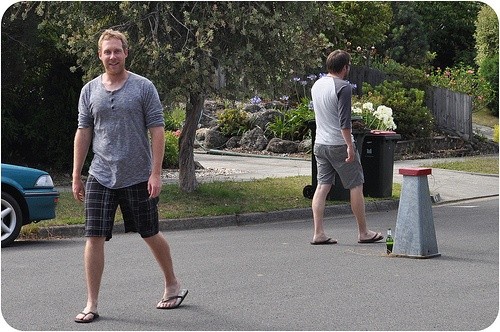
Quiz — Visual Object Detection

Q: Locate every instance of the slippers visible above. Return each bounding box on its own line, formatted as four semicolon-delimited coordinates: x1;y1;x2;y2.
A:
74;312;99;323
310;237;338;245
156;289;188;309
358;232;383;243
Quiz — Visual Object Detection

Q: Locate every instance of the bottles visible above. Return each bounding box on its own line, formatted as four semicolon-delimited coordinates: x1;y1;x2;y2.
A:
386;228;394;254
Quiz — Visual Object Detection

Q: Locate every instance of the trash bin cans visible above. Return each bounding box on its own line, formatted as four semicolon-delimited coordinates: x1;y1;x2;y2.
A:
303;119;371;201
361;130;401;198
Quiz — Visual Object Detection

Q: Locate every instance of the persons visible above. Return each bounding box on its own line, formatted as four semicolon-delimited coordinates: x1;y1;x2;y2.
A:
311;49;383;245
72;29;190;323
